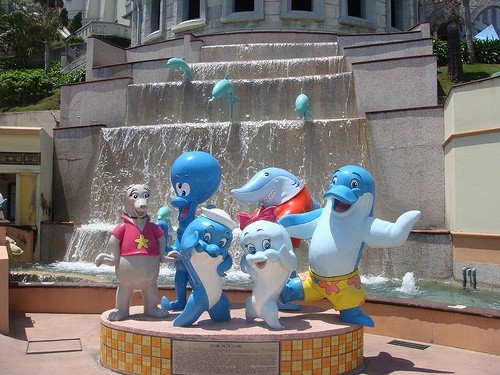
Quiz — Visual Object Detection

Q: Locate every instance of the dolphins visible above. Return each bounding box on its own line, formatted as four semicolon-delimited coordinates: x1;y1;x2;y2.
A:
294;88;312;120
210;74;239;123
168;57;192;84
